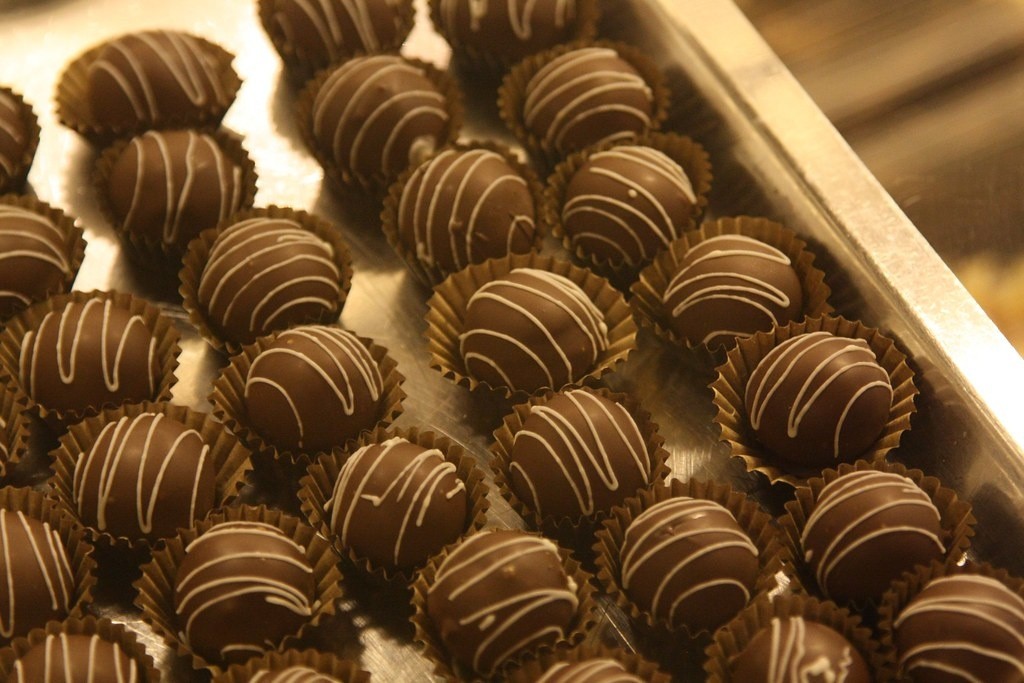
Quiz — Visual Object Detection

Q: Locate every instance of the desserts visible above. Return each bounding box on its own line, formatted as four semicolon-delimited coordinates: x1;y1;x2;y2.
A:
0;0;1024;683
